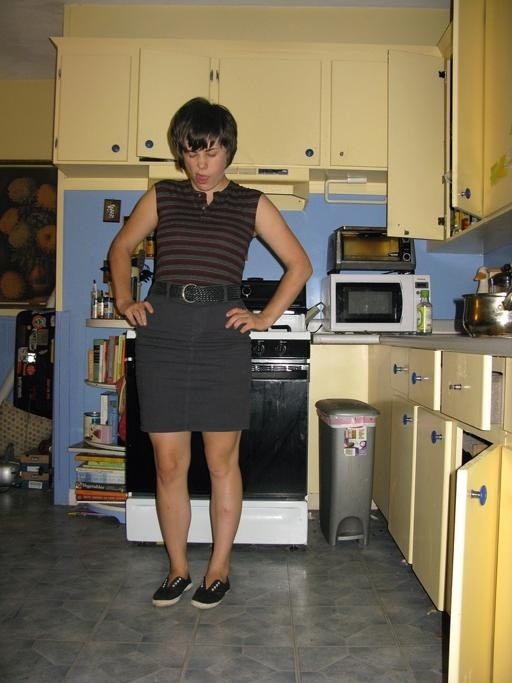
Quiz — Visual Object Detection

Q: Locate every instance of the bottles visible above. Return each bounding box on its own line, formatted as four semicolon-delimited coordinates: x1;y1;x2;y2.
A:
415;288;433;335
89;278;115;320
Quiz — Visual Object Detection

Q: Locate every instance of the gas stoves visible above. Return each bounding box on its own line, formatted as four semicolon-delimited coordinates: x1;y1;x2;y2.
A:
236;277;311;339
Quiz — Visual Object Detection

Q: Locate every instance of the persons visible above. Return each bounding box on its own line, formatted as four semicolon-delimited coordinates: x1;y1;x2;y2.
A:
104;96;315;610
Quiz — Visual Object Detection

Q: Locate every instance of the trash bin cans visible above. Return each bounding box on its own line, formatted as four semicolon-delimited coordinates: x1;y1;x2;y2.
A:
315;398;381;546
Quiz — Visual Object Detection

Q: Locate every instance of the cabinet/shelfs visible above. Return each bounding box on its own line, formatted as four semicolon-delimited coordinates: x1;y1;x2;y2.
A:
70;317;134;522
379;0;511;253
367;340;512;683
47;36;214;179
214;39;444;193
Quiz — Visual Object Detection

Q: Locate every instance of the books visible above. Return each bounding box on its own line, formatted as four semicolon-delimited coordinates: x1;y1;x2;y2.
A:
72;332;128;506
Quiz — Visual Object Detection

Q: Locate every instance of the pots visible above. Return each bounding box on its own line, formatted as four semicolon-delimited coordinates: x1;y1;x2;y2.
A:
460;289;512;339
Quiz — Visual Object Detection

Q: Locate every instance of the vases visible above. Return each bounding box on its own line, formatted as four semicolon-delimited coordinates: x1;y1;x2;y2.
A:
0;165;63;316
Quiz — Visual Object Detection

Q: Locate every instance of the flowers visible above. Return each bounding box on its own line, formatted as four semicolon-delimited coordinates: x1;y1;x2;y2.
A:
1;177;56;300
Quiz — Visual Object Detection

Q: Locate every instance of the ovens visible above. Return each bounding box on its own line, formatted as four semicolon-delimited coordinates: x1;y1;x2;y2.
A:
124;342;311;510
328;224;416;275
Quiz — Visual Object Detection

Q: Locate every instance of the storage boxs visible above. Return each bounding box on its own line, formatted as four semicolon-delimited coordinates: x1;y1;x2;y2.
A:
19;450;50;490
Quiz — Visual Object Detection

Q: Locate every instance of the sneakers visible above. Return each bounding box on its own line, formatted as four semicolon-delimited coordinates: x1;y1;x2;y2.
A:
191;573;231;610
150;574;193;607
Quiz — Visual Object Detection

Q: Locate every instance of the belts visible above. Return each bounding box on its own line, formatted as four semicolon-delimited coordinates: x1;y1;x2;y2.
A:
149;281;242;303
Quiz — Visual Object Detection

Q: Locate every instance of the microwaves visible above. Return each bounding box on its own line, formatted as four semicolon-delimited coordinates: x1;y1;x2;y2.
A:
319;270;432;334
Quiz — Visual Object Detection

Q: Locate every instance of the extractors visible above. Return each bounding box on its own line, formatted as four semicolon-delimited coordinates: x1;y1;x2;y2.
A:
144;161;312;213
